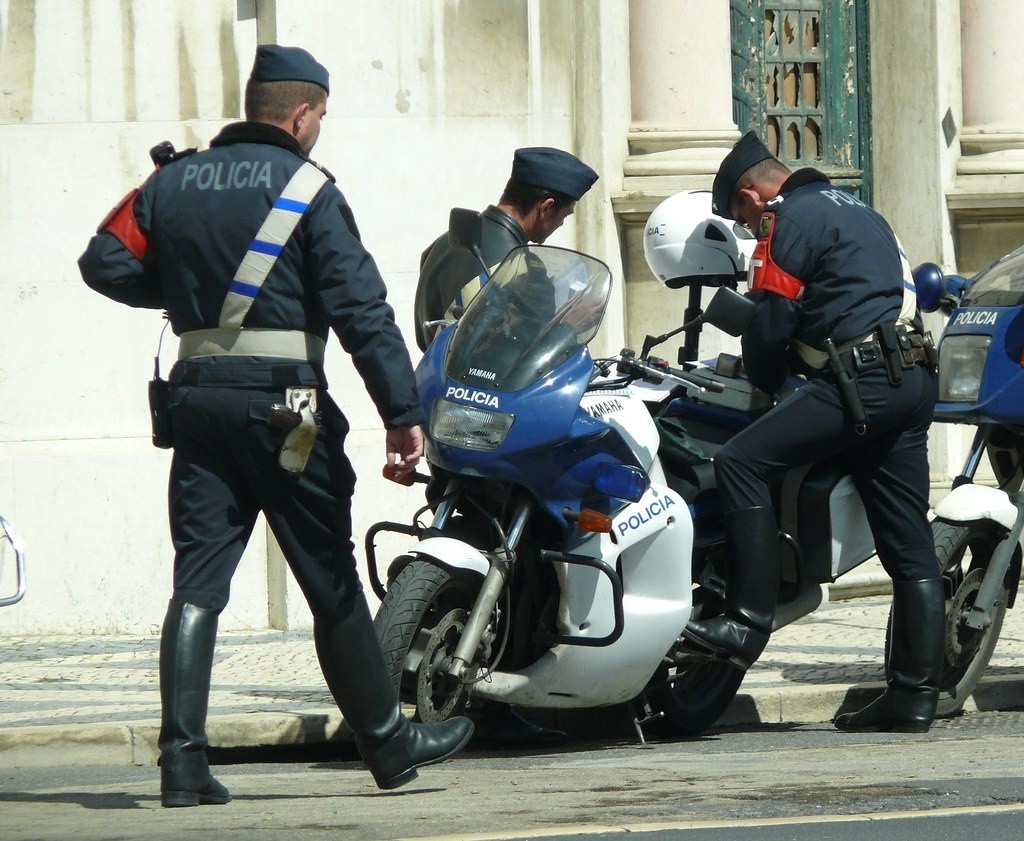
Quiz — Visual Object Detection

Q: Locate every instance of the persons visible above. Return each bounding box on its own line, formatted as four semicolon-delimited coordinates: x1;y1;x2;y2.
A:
683;131;944;733
73;46;474;809
415;148;609;359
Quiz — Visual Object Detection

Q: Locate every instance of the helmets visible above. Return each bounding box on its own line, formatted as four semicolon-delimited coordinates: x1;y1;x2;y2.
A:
643;189;761;289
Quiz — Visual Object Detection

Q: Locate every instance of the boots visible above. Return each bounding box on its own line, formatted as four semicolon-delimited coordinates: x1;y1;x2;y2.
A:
682;505;782;670
157;598;233;806
834;578;946;733
313;595;475;789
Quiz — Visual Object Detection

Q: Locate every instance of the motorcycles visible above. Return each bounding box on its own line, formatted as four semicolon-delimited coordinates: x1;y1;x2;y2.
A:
367;209;879;749
879;243;1024;717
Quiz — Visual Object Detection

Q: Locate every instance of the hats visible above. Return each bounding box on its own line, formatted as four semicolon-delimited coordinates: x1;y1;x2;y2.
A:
511;147;599;201
712;130;774;220
251;44;330;97
